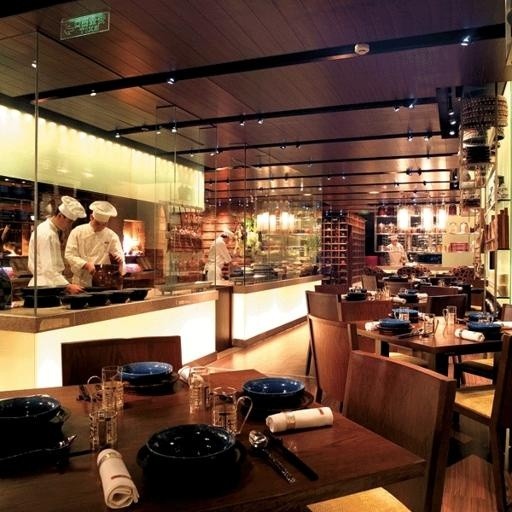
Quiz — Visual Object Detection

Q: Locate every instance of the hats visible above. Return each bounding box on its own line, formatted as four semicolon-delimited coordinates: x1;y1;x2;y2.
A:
88;200;118;223
57;196;86;221
222;228;235;240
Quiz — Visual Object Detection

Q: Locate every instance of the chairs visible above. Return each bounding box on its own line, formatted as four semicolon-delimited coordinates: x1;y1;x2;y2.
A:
456;302;510;389
338;349;456;511
303;263;512;373
454;334;510;511
457;32;474;49
237;119;265;128
393;152;441;205
452;172;462;190
446;89;465;138
305;312;360;411
58;334;187;387
188;143;347;194
392;98;416;113
405;129;432;143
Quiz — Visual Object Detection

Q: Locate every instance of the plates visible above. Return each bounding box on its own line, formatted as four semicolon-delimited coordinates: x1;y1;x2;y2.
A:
457;311;501;335
1;407;71;430
136;446;246;483
235;392;315;421
124;373;180;389
374;302;421;334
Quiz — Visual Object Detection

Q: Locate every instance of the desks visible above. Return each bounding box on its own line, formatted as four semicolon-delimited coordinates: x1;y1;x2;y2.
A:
341;315;502;373
1;365;427;512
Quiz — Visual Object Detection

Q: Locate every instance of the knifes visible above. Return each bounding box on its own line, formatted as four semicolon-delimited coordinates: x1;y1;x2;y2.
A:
262;430;320;480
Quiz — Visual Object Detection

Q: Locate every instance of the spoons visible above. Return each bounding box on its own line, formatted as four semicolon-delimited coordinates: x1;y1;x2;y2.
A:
249;429;296;485
1;435;80;460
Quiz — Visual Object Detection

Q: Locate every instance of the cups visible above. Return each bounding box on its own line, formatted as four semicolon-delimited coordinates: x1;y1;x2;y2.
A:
188;365;211;416
424;313;439;337
84;364;125;451
412;273;416;281
442;305;457;326
212;384;253;435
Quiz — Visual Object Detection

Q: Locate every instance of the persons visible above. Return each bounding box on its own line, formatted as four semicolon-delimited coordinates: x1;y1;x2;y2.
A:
64;200;126;287
386;235;408;268
28;195;87;294
203;229;238;281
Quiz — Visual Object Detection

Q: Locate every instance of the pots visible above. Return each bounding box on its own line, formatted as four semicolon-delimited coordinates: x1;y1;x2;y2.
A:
91;263;123;290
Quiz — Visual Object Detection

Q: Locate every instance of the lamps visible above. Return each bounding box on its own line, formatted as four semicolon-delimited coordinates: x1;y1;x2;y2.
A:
31;55;181;141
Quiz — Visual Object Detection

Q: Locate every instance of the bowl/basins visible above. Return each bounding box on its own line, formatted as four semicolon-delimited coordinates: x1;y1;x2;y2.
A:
62;287;152;309
117;361;173;384
346;288;368;300
242;377;304;407
145;424;234;469
399;289;418;302
0;395;62;427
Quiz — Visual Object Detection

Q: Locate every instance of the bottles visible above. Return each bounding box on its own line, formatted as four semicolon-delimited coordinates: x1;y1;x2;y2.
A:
487;208;509;251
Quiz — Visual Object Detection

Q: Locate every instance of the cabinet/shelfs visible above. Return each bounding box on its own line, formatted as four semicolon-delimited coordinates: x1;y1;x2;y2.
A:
313;211;368;289
